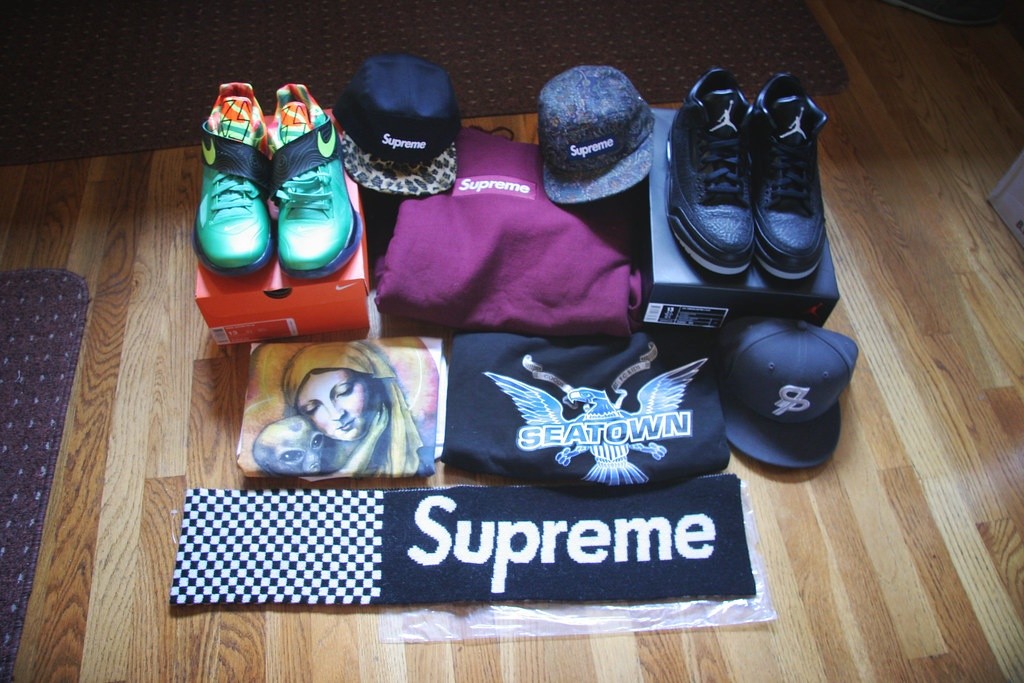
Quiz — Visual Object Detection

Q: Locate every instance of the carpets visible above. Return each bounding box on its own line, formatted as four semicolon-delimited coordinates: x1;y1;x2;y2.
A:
0;0;852;167
0;267;89;683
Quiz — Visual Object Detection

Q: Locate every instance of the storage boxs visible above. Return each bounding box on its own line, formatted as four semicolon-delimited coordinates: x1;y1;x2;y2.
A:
195;108;370;346
629;110;841;346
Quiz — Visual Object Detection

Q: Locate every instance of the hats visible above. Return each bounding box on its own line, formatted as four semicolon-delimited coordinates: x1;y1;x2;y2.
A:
537;64;655;205
708;316;859;468
332;55;463;197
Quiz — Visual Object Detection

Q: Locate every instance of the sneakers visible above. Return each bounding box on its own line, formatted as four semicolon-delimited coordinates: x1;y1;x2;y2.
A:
746;71;829;280
192;82;273;277
665;67;756;275
265;82;362;280
